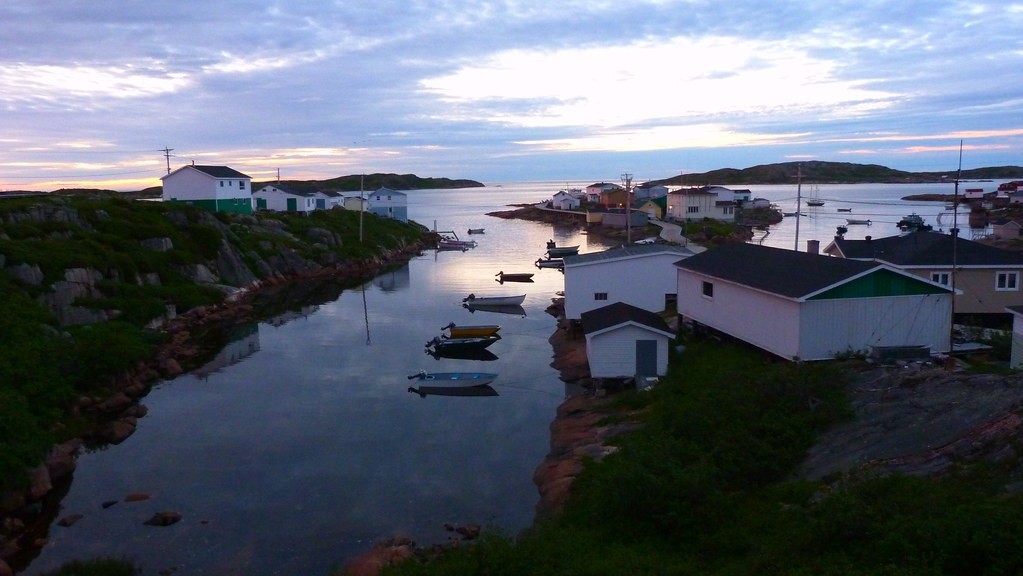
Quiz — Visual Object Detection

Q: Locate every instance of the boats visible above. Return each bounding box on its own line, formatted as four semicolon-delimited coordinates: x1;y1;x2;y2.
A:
469;228;484;233
437;240;466;248
408;369;500;391
440;322;502;337
846;219;871;224
462;293;526;306
432;336;499;351
549;245;579;255
497;271;534;280
806;199;824;206
537;260;564;266
